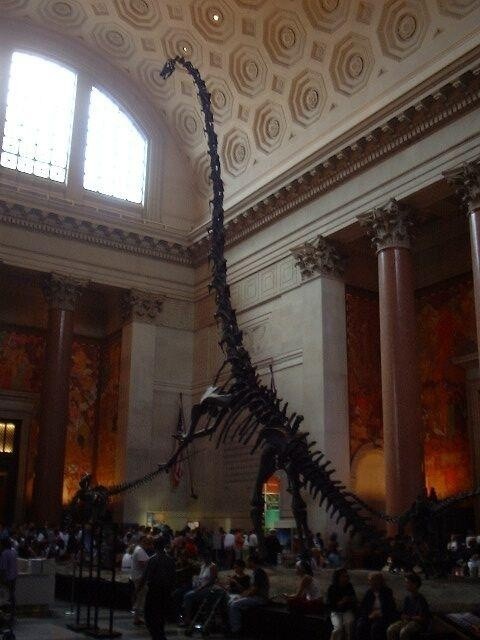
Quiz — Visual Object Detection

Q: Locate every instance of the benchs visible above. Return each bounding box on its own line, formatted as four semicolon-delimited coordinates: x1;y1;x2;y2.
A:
55;570;374;640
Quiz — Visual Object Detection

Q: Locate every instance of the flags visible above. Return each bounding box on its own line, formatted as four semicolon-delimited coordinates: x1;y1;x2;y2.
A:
170;407;186;487
